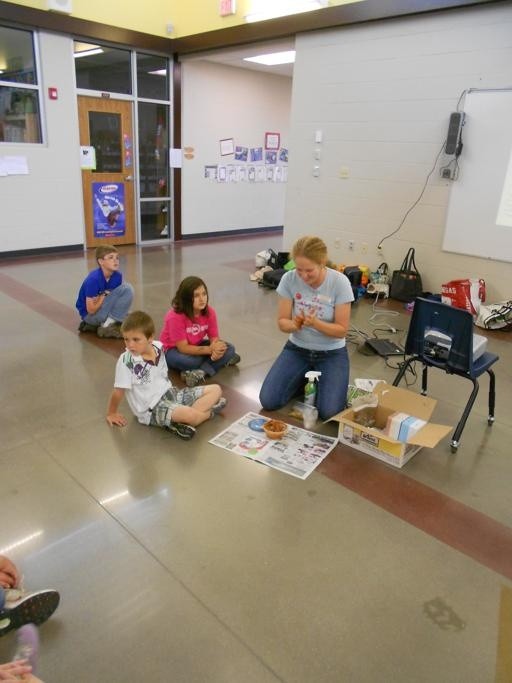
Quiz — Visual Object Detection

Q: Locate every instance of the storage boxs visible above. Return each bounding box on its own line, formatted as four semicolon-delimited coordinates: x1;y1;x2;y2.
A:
322;381;454;469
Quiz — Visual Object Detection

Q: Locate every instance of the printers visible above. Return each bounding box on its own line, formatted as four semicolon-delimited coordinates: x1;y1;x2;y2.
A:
423;331;488;364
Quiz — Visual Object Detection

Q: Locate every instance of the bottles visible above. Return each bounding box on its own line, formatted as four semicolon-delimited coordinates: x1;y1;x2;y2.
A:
292;401;318;419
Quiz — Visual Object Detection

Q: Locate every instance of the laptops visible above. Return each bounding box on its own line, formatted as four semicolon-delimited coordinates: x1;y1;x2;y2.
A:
349;321;406;357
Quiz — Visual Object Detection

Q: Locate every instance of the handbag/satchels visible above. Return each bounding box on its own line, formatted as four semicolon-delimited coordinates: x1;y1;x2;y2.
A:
441;278;485;322
342;266;361;303
475;300;512;331
256;250;280;267
370;263;389;287
263;269;286;289
390;248;422;302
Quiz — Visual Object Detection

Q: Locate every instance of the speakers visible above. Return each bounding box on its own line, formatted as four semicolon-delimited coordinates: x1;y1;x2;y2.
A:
444;112;466;158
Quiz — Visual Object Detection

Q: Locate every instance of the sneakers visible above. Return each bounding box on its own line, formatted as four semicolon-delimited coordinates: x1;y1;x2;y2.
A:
226;354;240;366
214;399;226;413
164;422;196;440
7;624;40;678
0;589;60;636
180;369;201;387
98;326;122;338
79;321;96;332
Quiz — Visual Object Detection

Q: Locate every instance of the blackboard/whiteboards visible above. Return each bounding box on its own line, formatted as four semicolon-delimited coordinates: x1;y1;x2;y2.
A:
440;89;512;267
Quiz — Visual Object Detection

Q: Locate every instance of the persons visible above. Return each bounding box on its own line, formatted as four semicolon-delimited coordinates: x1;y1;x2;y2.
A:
0;623;44;683
259;237;355;421
95;194;124;227
162;276;241;386
77;246;134;340
106;311;228;441
0;554;62;635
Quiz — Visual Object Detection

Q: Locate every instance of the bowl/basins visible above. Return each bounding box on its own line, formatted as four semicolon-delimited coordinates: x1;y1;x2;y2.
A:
264;421;288;440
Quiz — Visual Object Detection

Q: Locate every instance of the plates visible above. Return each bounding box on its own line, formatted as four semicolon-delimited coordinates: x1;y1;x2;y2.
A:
248;418;267;432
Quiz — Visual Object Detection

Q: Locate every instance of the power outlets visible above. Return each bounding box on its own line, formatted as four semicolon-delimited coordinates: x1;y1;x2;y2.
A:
315;131;322;142
347;240;354;250
314;149;321;160
360;243;368;253
376;246;383;251
313;166;319;177
334;238;342;248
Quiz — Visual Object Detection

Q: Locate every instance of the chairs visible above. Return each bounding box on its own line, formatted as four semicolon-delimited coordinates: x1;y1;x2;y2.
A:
392;296;500;455
